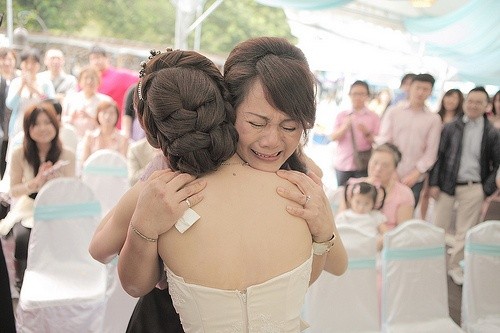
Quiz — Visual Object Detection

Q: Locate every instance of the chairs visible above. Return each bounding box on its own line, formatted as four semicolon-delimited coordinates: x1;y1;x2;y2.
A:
15;179;110;333
381;219;463;333
81;150;131;212
461;220;500;333
301;226;379;333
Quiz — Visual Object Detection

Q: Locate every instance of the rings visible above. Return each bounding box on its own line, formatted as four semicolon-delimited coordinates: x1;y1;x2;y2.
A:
185;198;191;208
304;196;311;208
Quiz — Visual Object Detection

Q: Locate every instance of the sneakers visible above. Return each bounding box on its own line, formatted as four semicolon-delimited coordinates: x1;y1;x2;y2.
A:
448;266;465;286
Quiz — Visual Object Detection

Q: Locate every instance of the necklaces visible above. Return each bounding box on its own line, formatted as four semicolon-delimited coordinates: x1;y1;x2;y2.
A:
221;160;249;166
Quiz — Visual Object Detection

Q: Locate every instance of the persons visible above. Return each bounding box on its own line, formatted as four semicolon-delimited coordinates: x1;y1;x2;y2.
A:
437;89;464;126
89;49;333;332
36;48;77;99
333;182;392;252
75;102;130;177
0;46;22;179
40;99;79;154
331;81;381;187
127;135;164;188
5;48;56;164
87;45;140;130
340;143;414;231
425;87;500;286
62;66;116;136
0;103;79;294
383;74;418;116
67;69;116;137
372;73;443;208
121;82;146;144
485;89;500;129
117;37;348;333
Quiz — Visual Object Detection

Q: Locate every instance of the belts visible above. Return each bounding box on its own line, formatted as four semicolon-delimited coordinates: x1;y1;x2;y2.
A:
456;181;478;186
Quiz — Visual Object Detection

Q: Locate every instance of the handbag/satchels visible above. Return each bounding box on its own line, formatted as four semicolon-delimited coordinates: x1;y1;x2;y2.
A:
349;112;371;172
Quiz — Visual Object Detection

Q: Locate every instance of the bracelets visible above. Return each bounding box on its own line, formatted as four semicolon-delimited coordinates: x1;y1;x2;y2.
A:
312;233;337;255
129;221;158;242
